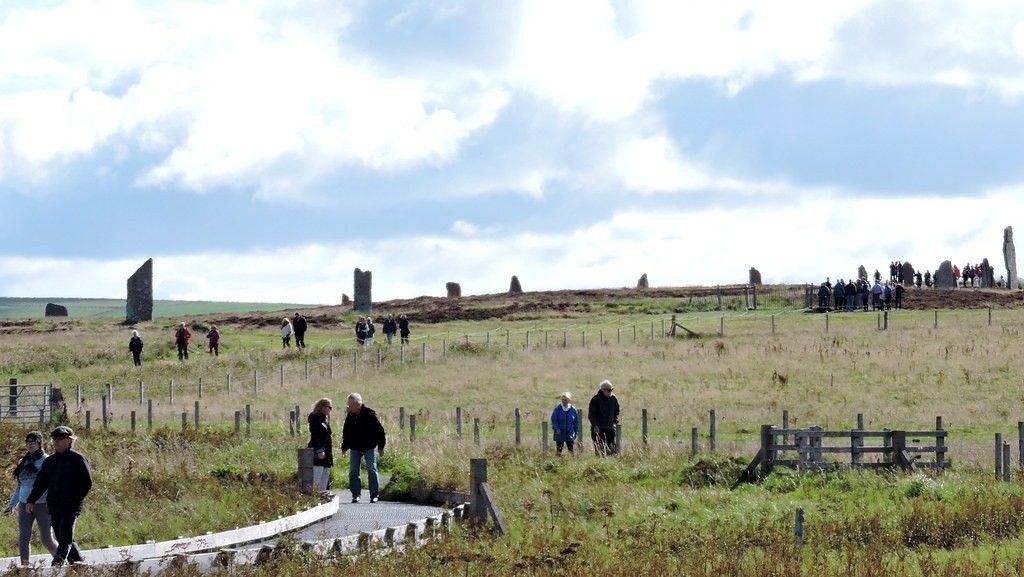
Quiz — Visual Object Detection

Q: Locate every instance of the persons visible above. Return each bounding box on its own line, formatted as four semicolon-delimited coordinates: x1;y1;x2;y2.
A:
873;268;882;285
890;260;905;287
925;270;933;289
127;331;144;366
279;317;292;350
912;270;924;291
551;392;579;457
382;314;398;344
292;312;308;350
6;426;92;568
355;315;376;350
816;277;906;312
206;324;221;357
176;323;192;361
400;314;411;346
587;382;620;457
996;276;1008;289
343;394;388;503
952;263;984;289
307;397;335;495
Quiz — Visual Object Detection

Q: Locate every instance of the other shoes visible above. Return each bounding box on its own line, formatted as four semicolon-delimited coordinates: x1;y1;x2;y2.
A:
351;496;360;503
370;496;379;503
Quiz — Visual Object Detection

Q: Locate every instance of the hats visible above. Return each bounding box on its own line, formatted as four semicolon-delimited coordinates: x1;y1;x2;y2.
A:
27;431;42;444
49;426;73;438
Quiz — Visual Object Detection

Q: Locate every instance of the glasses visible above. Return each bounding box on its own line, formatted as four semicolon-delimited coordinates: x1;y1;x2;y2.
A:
326;405;333;410
26;439;37;445
603;388;614;392
51;435;69;441
